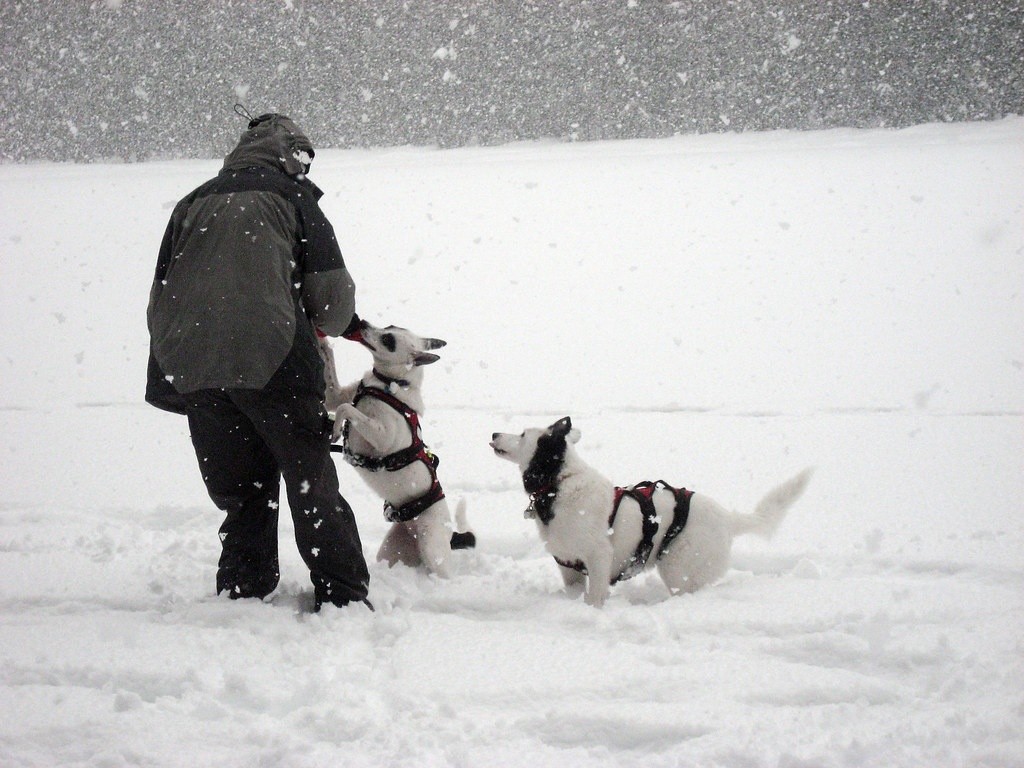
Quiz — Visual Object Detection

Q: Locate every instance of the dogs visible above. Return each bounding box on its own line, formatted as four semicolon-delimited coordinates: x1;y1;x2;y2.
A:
308;309;475;587
487;415;816;612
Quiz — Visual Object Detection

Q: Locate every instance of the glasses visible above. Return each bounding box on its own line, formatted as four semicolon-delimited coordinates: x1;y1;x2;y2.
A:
297;159;311;174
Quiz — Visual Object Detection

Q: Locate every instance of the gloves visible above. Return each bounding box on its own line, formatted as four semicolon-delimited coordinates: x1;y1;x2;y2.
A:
344;315;367;339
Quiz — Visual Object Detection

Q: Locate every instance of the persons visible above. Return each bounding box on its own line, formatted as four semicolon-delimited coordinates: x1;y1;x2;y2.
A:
144;114;369;614
